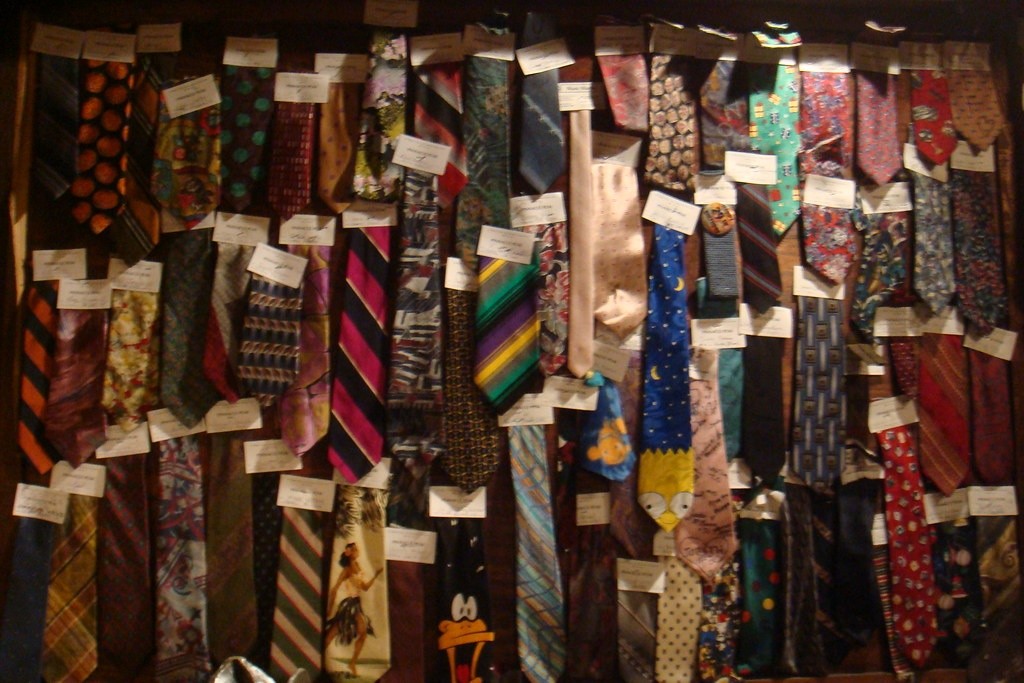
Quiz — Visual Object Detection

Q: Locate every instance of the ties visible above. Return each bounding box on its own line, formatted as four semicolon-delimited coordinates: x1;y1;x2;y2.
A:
0;17;1024;683
